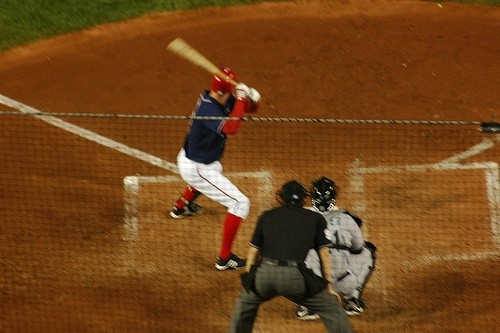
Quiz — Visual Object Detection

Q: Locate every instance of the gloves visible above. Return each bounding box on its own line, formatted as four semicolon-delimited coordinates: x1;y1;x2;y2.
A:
246;86;262;106
234;81;251;103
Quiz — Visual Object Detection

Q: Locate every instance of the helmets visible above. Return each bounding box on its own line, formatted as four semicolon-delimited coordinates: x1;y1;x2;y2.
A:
308;176;340;213
275;179;311;208
208;66;239;98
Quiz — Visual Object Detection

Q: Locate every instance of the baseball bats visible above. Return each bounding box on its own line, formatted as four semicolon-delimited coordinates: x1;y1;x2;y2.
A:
167;37;256;101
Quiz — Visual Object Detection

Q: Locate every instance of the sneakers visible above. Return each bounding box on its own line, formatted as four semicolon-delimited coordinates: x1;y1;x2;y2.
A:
169;205;197;219
295;305;321;321
214;252;247;271
343;299;367;315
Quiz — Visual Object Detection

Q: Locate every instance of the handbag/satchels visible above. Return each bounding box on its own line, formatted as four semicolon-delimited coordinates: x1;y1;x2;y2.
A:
239;264;259;296
298;263;330;297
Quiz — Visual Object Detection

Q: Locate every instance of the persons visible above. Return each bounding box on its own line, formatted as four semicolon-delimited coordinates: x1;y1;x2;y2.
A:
293;175;377;320
170;67;263;271
228;179;359;333
